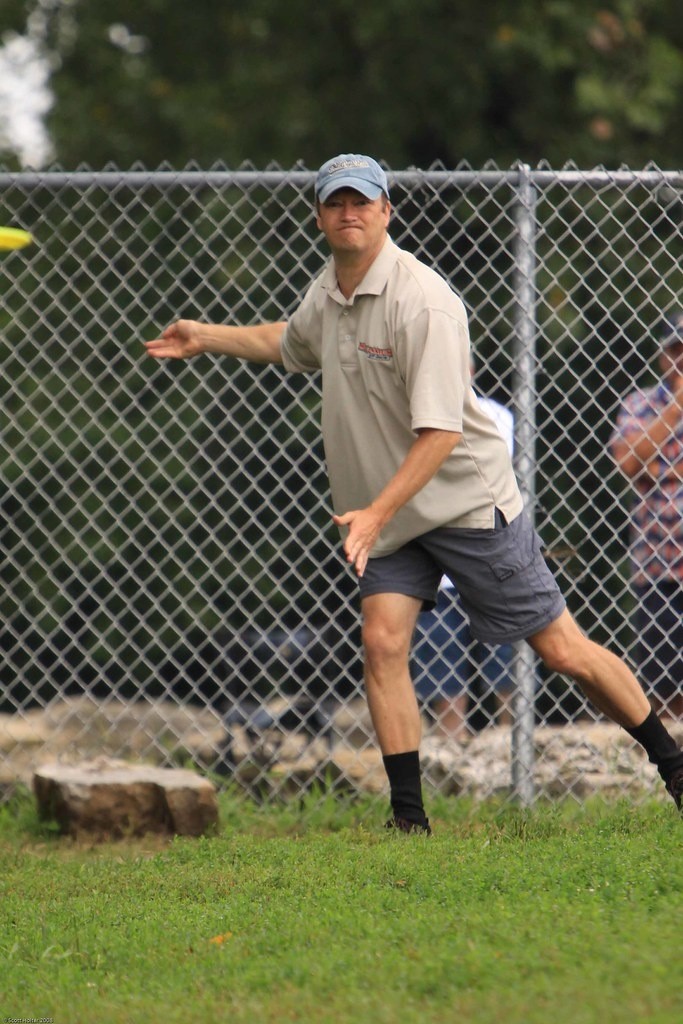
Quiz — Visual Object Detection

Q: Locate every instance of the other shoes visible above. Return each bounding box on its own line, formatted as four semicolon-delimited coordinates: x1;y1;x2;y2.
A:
382;819;432;840
665;763;682;815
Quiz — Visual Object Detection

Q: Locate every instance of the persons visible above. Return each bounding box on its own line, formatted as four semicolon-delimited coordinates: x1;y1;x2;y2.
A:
415;593;517;731
140;153;683;835
613;337;683;701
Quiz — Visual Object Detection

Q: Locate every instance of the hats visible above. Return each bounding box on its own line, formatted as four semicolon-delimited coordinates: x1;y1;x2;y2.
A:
658;315;682;344
315;153;390;201
